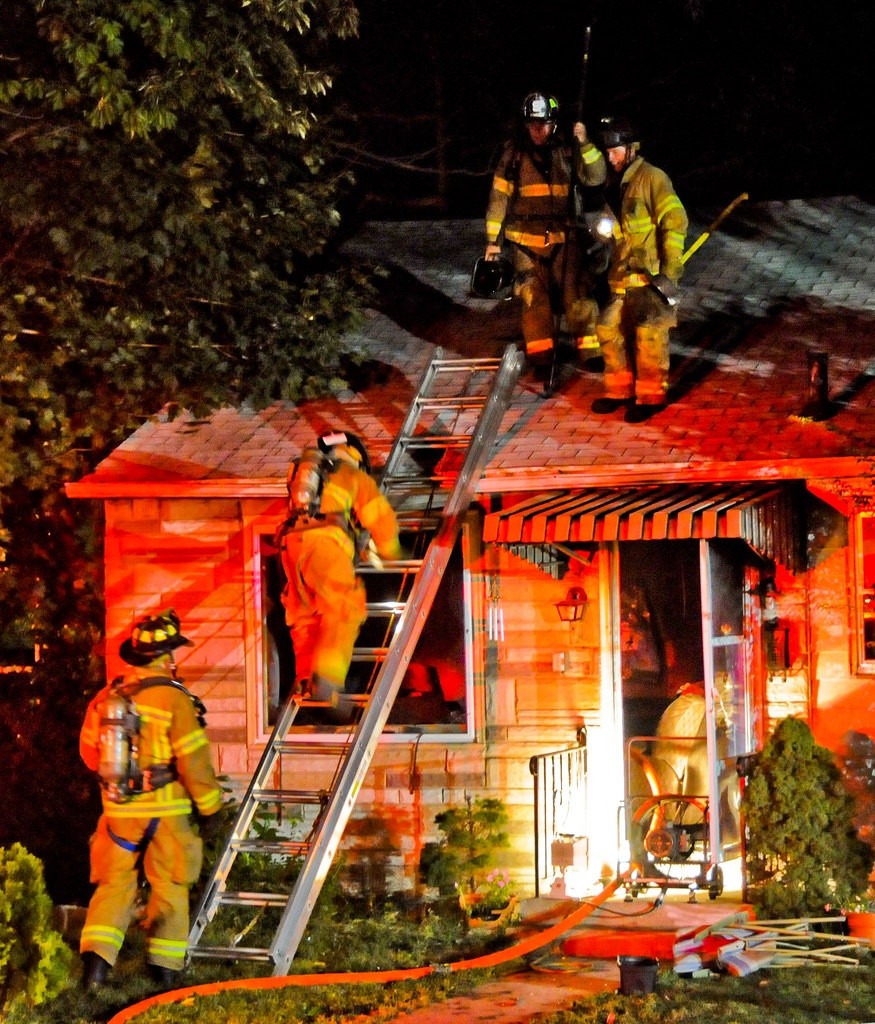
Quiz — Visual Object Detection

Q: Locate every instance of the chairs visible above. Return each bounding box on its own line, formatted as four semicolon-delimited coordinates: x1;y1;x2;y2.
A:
673;912;871;977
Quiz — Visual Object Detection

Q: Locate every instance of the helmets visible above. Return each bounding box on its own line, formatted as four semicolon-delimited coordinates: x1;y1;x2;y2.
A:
317;431;371;474
120;614;194;665
602;121;637;150
520;91;559;123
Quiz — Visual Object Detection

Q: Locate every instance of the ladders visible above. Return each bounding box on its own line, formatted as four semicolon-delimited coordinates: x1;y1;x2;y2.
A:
179;338;532;980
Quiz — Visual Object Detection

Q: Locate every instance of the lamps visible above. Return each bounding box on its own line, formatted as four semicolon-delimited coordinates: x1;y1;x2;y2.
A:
743;578;784;630
553;588;588;622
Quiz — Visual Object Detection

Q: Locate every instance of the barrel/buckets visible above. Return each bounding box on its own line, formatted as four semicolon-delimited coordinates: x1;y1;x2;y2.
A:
616;954;659;997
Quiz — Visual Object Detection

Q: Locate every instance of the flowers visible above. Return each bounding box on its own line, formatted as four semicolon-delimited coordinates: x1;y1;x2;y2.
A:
822;877;875;914
453;869;512;919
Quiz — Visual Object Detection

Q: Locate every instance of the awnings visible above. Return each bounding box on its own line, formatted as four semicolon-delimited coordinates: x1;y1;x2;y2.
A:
484;490;797;576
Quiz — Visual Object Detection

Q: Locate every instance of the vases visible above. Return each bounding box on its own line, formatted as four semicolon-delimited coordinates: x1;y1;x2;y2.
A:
458;893;518;929
847;912;875;950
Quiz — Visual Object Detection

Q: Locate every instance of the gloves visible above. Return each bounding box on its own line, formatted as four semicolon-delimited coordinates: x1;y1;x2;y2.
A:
197;809;228;839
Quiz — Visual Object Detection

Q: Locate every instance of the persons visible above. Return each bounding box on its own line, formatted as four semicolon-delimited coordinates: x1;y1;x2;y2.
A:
585;113;693;421
486;90;610;386
80;614;240;994
273;431;407;720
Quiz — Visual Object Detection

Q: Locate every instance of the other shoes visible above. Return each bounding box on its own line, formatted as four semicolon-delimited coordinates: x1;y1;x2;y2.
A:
592;395;636;414
585;356;605;373
78;953;107;988
534;361;552;380
149;966;177;985
624;403;667;423
296;677;337;700
